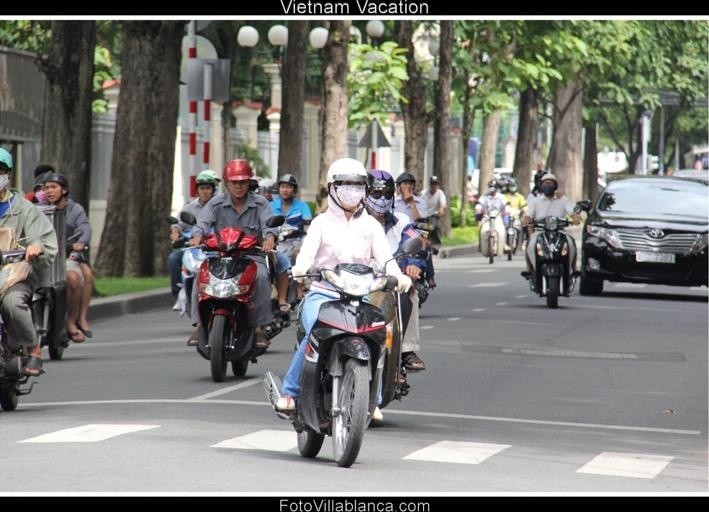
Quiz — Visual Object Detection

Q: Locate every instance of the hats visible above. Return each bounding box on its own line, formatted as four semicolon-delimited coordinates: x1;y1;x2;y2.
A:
430;175;441;185
0;147;14;170
31;173;48;193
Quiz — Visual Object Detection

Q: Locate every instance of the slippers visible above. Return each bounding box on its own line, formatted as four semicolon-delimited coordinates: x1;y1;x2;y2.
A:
22;354;43;376
278;303;291;315
67;326;85;343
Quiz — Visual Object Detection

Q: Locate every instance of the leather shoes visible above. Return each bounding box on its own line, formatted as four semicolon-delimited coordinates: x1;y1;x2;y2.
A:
76;319;93;338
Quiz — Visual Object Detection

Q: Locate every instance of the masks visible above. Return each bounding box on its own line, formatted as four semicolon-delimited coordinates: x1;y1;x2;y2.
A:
508;186;517;193
34;191;49;205
365;195;394;217
488;187;496;192
334;184;366;208
542;185;556;196
0;173;10;192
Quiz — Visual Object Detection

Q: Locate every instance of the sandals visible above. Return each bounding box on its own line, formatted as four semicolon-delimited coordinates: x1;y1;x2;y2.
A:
401;351;427;371
394;372;407;385
253;330;269;350
187;329;200;346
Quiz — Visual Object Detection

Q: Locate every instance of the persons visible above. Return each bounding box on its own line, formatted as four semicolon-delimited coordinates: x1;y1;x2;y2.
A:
167;157;449;420
465;169;581;279
0;146;94;378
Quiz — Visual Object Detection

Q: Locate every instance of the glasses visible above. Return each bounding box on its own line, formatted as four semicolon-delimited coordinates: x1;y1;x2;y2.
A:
368;190;393;200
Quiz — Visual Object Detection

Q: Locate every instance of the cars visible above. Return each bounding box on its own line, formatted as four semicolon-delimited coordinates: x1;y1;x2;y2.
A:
577;175;709;293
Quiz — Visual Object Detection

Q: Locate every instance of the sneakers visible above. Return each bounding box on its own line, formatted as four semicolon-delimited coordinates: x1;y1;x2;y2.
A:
276;394;296;411
427;279;439;288
371;406;384;421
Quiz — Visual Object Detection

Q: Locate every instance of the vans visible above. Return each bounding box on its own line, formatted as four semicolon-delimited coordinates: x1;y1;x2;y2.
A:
472;167;547;189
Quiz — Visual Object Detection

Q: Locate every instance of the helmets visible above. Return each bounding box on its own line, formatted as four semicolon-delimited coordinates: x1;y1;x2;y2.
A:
195;173;216;188
44;172;69;188
200;169;222;182
397;172;416;186
326;158;368;185
534;170;548;184
540;173;558;189
488;180;498;187
508;178;519;185
366;169;396;195
278;174;298;194
222;158;254;184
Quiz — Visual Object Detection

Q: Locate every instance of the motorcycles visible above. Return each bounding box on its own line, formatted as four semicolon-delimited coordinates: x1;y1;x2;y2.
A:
160;213;314;324
383;214;442;304
470;194;525;263
519;209;581;309
264;264;413;467
0;226;89;411
176;210;285;382
